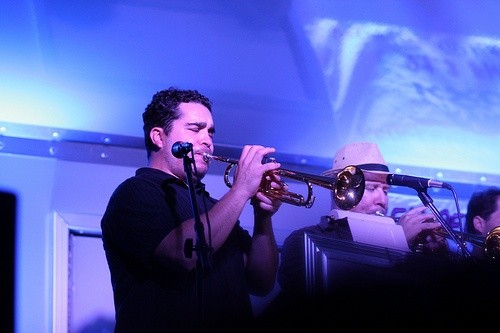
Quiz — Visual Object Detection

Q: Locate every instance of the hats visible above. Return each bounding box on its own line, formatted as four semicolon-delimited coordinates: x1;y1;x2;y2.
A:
321;141;393;182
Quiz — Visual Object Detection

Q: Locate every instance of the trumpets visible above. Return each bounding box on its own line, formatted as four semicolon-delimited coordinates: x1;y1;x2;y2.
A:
375;210;500;264
198;152;366;211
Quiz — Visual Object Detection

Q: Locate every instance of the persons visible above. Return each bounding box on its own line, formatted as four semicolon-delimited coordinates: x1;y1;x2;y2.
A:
464;187;500;256
279;142;443;291
100;87;289;333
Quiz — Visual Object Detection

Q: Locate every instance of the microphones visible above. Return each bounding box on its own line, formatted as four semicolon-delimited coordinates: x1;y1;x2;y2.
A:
387;173;452;189
171;140;193;158
